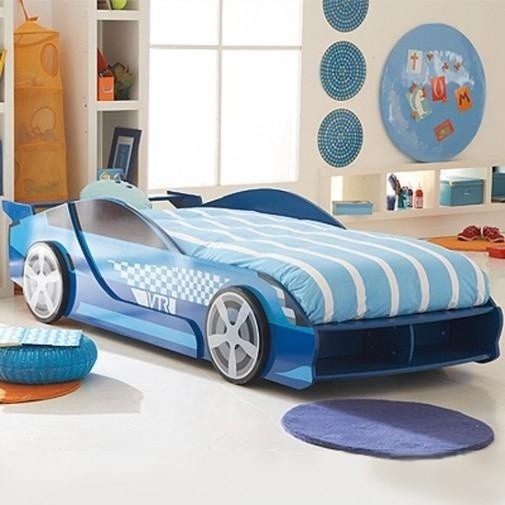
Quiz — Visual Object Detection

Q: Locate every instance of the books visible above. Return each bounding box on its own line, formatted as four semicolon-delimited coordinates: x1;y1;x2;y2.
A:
0;326;85;348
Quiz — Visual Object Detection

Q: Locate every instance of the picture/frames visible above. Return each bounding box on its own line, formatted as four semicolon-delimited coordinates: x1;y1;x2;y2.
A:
107;127;143;181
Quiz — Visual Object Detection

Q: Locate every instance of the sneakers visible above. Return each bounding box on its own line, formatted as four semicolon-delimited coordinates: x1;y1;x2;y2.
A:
484;227;505;242
457;225;481;241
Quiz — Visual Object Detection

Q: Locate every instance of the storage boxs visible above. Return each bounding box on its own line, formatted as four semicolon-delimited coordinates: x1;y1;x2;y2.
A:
441;176;484;205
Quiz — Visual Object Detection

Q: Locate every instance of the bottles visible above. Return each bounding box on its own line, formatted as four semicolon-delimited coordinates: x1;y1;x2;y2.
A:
407;182;423;208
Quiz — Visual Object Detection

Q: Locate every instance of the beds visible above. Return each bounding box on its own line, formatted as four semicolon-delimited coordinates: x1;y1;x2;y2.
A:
0;186;505;390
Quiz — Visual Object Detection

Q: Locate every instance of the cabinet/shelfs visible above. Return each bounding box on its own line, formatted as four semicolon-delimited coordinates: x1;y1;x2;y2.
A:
51;0;151;199
0;0;14;298
317;157;505;223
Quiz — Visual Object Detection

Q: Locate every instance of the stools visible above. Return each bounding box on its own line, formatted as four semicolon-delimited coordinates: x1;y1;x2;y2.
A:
0;334;98;385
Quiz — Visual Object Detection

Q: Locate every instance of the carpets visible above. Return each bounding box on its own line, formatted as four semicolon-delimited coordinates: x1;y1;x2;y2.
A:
426;237;505;252
280;398;496;461
0;382;79;404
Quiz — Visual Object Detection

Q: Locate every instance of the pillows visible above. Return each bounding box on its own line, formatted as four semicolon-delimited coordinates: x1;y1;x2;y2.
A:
79;179;153;210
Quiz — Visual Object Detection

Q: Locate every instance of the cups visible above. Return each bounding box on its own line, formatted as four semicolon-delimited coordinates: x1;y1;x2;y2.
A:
387;194;397;209
